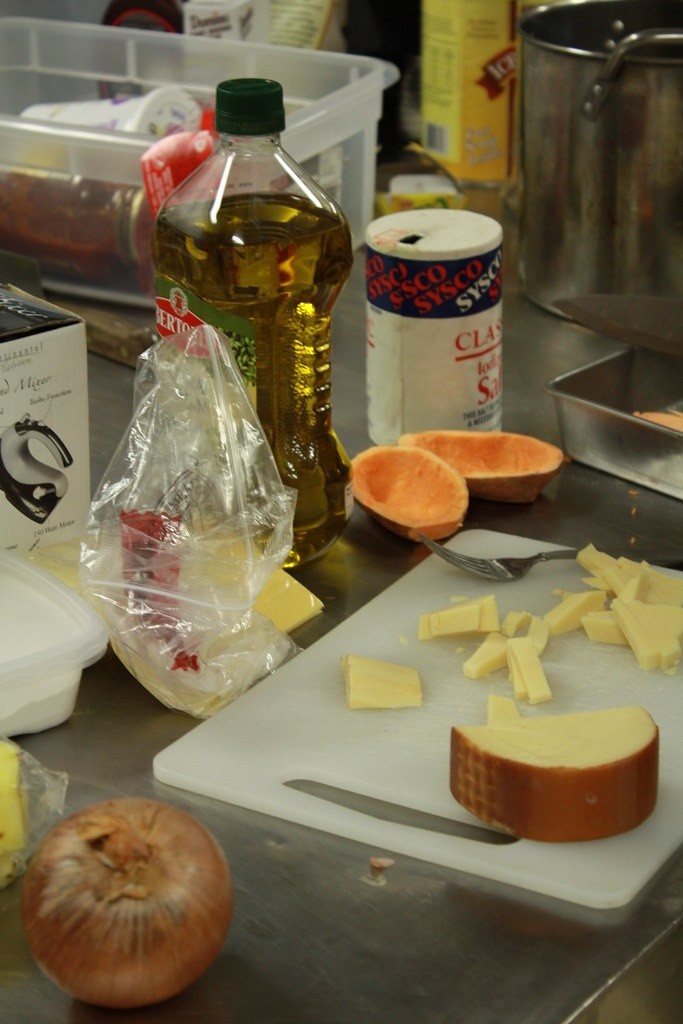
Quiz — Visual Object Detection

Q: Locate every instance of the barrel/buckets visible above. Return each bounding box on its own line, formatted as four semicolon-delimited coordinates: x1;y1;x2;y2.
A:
510;0;683;357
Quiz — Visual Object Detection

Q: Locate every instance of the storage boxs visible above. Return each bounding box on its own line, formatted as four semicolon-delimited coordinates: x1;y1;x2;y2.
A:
0;16;403;307
0;281;92;550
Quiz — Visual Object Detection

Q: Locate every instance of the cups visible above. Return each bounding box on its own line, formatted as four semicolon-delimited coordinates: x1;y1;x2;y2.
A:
22;78;200;139
362;209;504;444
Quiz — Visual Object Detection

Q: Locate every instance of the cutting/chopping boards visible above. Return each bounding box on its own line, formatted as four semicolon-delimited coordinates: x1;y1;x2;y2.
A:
153;529;683;909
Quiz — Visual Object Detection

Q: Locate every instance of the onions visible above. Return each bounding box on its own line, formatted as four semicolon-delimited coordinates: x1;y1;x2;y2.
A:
22;796;231;1007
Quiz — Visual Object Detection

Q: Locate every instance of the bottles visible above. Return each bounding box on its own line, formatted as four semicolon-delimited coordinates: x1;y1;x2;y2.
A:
420;0;521;186
0;166;151;290
151;78;353;568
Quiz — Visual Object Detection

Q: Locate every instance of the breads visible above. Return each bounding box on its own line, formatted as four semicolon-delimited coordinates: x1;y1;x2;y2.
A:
446;704;661;841
340;544;683;720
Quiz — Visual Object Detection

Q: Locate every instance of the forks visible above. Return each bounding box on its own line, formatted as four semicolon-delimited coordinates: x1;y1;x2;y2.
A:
417;531;683;582
